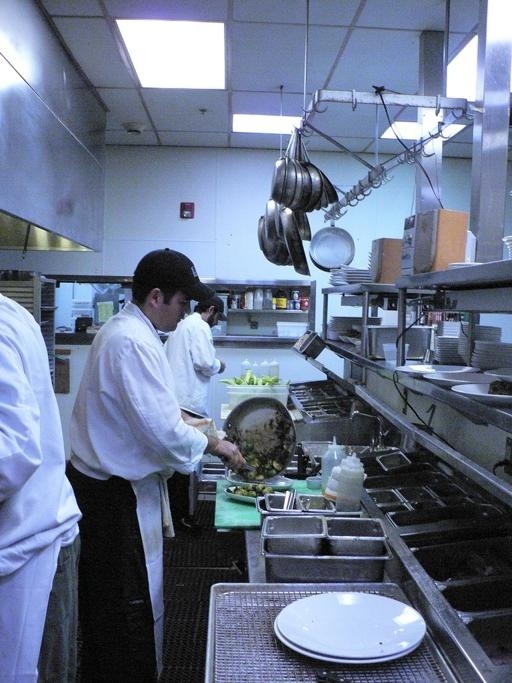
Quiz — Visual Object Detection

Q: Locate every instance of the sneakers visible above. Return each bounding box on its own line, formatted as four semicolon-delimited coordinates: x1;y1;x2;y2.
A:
175;515;201;537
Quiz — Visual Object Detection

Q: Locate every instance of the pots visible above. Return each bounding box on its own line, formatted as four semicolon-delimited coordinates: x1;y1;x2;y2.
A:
200;398;298;482
257;129;357;276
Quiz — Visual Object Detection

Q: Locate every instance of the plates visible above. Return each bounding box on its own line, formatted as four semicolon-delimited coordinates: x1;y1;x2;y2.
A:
225;472;294;490
268;592;430;668
223;482;267;506
395;322;511;404
448;262;483;270
328;269;372;286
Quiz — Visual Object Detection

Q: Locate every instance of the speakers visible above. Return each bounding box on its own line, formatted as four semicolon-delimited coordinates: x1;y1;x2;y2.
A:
413;209;470;275
371;238;402;284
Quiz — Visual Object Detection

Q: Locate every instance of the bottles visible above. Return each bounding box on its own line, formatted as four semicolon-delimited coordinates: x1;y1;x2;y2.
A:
240;287;287;312
321;435;365;509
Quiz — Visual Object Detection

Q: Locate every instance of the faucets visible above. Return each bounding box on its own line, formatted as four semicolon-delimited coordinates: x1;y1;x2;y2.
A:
350;411;390;449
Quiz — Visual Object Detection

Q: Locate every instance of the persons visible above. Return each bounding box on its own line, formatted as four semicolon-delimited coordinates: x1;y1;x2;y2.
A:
64;244;250;682
0;290;85;682
164;296;231;537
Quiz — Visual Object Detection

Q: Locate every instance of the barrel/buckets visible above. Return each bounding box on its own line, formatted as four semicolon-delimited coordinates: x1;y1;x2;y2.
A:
244;287;254;310
263;288;272;309
253;288;264;310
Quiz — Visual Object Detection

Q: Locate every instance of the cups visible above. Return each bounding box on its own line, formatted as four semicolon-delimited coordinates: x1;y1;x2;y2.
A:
286;290;310;313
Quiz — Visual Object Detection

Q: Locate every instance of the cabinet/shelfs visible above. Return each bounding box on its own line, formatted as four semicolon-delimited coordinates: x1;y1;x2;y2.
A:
200;277;315;342
321;259;512;434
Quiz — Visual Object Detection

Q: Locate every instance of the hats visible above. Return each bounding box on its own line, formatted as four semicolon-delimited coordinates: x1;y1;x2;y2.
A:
133;249;215;302
198;296;227;321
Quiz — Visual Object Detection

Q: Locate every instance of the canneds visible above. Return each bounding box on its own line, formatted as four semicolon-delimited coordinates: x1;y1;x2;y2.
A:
230;294;242;309
272;290;309;310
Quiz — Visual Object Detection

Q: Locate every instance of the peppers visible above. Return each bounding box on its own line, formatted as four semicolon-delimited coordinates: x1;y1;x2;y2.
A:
218;371;292;386
